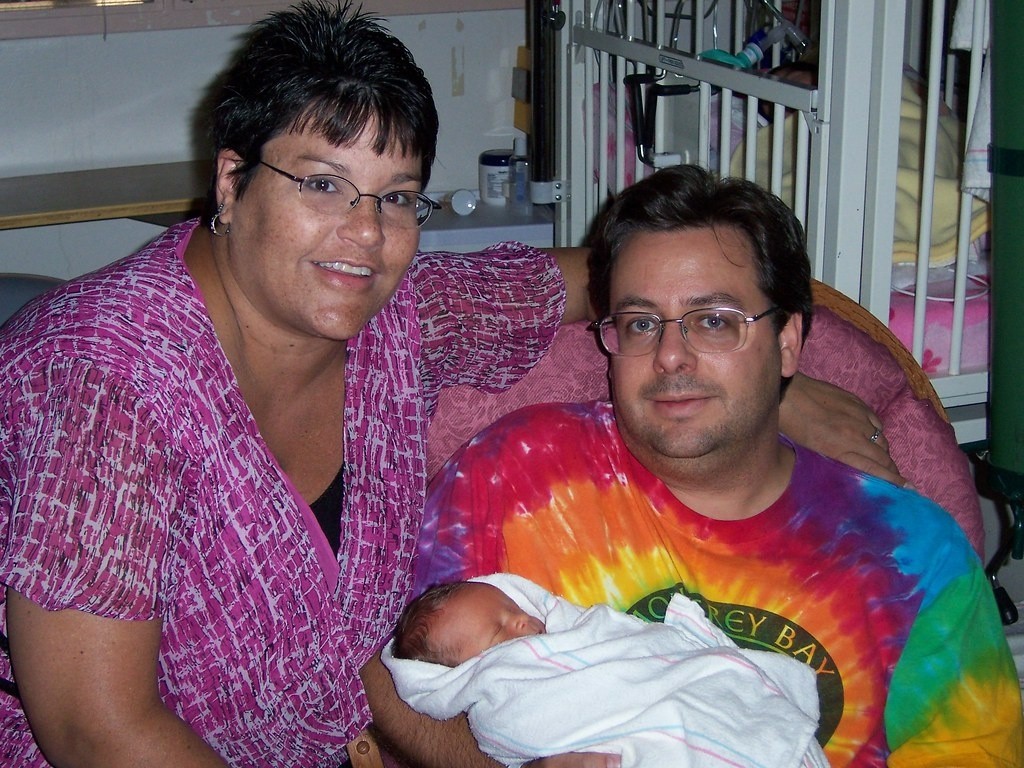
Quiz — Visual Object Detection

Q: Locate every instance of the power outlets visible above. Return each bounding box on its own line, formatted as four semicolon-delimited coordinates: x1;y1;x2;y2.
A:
510;67;529;103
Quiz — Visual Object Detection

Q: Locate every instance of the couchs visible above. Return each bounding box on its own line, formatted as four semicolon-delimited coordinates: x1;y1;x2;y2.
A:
348;280;986;768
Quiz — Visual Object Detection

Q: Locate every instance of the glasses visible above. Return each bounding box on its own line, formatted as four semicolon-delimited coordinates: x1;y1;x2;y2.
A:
257;159;442;229
590;304;782;357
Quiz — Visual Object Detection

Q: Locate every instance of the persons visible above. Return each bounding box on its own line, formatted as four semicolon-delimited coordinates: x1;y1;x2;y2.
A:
0;0;906;768
379;572;835;768
350;166;1024;768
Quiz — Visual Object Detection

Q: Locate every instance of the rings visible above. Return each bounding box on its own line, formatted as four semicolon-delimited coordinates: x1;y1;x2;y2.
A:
872;427;882;443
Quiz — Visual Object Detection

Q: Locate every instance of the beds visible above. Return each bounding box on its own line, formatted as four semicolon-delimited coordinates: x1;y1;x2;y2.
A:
530;0;990;443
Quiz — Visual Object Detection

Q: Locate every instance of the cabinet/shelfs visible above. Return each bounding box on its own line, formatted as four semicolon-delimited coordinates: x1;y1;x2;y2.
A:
417;197;553;252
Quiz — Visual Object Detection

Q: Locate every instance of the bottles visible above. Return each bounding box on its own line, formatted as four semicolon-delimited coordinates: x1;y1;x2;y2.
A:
509;136;533;218
